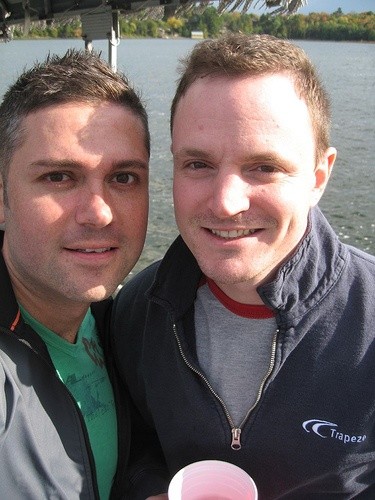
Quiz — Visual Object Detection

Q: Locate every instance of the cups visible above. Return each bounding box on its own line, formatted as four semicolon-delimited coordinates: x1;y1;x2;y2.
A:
168;460;259;500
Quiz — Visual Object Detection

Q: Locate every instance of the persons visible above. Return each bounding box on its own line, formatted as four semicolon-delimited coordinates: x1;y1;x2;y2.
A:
102;27;375;500
0;46;151;498
